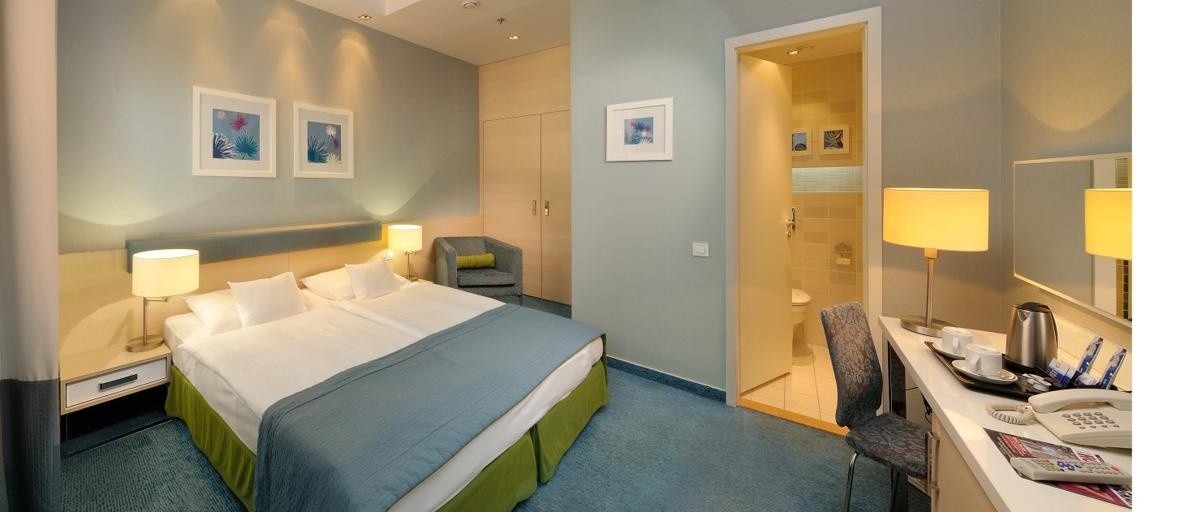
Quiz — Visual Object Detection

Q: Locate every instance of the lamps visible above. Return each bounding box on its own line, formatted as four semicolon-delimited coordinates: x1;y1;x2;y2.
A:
387;223;423;282
881;185;991;339
125;247;201;354
1084;187;1132;322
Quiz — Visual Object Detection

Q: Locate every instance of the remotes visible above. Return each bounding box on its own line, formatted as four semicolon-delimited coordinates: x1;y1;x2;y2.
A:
1009;456;1132;486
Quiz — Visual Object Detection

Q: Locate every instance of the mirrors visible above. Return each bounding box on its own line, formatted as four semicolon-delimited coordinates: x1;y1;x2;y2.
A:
1009;150;1132;331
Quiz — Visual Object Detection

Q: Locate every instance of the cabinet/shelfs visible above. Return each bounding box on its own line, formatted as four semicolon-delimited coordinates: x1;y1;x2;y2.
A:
482;108;572;308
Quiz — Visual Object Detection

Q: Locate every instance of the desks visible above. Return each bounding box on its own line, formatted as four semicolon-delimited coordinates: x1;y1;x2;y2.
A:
876;313;1131;512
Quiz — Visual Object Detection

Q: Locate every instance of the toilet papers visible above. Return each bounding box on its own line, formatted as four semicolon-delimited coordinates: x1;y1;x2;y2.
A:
836;258;851;266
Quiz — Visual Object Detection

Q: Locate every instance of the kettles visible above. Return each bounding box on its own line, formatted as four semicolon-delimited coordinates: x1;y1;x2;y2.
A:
1003;302;1059;374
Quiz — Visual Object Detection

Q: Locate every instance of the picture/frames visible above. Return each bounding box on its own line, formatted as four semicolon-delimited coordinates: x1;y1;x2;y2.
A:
291;99;354;180
818;123;850;154
605;96;675;162
190;84;278;178
792;127;813;157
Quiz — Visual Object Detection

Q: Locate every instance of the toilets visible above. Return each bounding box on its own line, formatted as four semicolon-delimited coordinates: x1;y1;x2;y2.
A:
792;288;811;329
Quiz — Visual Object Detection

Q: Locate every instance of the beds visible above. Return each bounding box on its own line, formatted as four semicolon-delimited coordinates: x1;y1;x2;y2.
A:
125;220;612;512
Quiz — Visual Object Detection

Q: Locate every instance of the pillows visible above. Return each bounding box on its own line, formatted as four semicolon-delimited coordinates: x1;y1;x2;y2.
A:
457;251;496;271
180;288;243;340
344;256;403;303
300;264;411;305
224;271;310;330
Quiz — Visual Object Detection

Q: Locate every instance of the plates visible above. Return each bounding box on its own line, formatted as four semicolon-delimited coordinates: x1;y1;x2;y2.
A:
951;358;1018;385
932;339;970;360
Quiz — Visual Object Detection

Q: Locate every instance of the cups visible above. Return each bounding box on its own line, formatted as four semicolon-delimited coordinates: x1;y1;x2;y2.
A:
970;349;1002;376
941;324;973;356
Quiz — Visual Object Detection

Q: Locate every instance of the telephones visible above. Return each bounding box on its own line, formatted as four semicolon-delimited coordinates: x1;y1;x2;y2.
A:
1028;387;1131;450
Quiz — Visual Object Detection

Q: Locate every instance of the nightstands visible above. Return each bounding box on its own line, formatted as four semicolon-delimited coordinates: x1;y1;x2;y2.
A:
418;278;435;285
59;336;180;457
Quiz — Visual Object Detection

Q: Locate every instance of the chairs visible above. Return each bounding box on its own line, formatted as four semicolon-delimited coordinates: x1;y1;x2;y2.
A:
433;235;524;307
817;302;931;511
